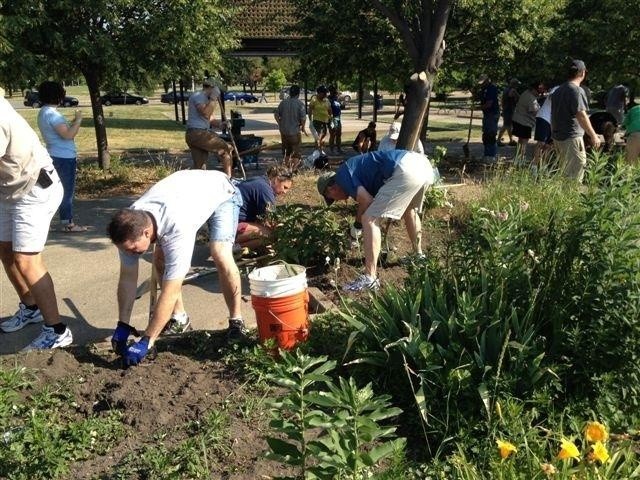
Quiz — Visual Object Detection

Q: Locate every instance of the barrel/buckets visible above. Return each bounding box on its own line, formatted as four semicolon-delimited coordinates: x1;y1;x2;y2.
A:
247;264;307;298
253;289;309;349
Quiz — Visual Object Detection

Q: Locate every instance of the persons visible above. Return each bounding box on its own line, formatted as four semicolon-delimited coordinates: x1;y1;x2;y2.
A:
38;80;88;232
231;163;293;265
185;80;426;176
316;150;435;291
106;169;247;369
0;93;74;353
470;60;640;185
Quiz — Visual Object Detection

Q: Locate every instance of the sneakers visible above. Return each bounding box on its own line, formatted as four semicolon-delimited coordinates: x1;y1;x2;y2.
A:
22;325;73;354
342;273;380;292
508;141;517;145
0;303;43;333
398;253;426;265
60;224;87;232
227;317;245;334
159;314;194;336
235;247;258;259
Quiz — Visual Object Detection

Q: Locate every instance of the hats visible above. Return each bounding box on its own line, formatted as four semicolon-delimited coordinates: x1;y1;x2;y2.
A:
388;121;401;140
317;171;336;208
477;73;488;84
568;59;589;72
202;77;226;91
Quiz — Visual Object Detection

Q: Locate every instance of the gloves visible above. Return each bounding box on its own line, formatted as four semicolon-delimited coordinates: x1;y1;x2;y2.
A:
349;223;362;240
218;119;232;131
116;337;155;367
110;323;131;356
209;86;221;100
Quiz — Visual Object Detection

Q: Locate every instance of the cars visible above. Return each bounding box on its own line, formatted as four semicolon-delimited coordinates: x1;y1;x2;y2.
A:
298;89;317;101
101;91;148;106
161;91;193;104
225;91;259;103
339;90;358;103
23;91;78;109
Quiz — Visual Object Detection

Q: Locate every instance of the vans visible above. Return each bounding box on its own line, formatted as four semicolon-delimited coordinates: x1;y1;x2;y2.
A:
280;90;289;99
